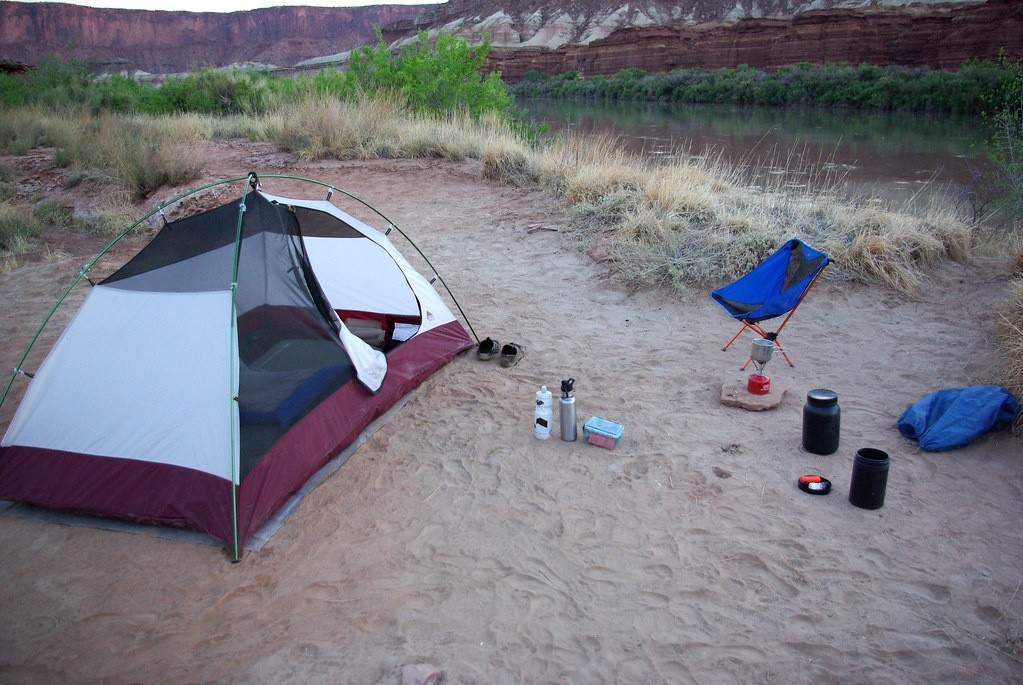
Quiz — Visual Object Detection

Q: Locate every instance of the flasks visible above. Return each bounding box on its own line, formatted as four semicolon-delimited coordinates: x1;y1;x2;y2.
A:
559;378;577;442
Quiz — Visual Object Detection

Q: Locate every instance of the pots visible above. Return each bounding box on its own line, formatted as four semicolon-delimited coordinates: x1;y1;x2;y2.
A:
750;338;774;362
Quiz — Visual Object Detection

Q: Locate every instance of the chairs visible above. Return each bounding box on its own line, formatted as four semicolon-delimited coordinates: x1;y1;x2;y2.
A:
710;238;835;372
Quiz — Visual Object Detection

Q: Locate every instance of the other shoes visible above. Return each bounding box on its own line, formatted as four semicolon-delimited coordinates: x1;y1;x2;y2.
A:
476;336;501;360
499;342;526;367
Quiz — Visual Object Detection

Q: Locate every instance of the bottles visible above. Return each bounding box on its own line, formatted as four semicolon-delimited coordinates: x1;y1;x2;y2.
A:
807;482;827;490
533;385;552;440
799;476;822;484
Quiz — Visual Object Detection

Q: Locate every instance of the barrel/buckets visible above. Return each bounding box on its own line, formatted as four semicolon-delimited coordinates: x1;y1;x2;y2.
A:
802;389;841;455
847;448;890;509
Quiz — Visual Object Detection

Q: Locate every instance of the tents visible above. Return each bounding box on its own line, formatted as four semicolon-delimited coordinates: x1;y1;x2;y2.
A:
0;172;481;563
